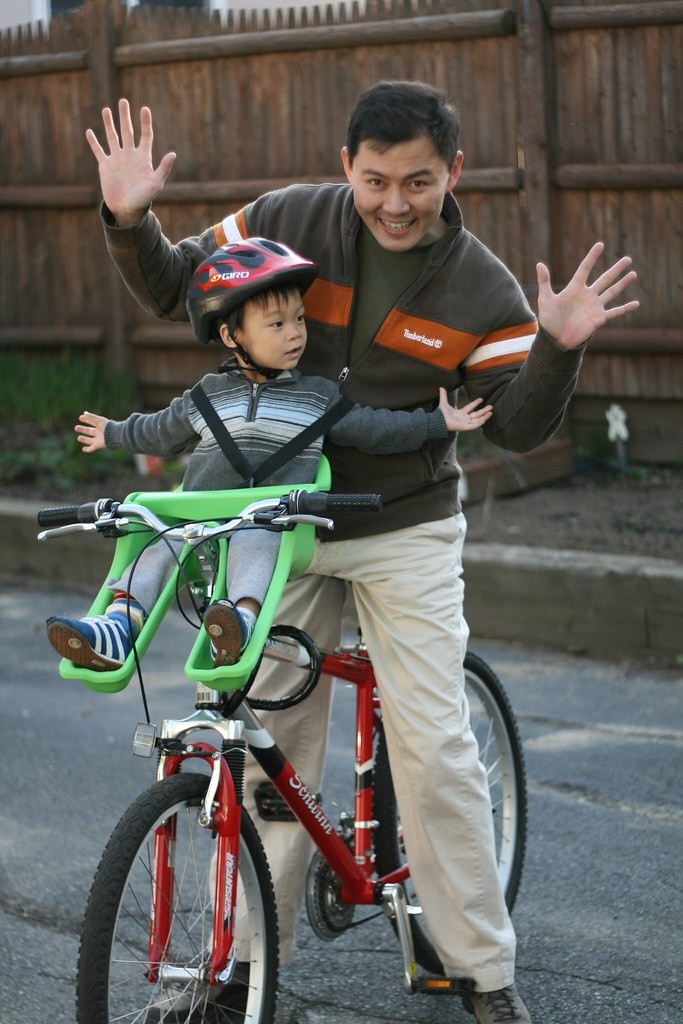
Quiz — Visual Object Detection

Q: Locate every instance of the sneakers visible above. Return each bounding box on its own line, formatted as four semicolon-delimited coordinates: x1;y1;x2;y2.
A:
43;610;139;671
203;598;249;669
145;962;252;1024
463;983;533;1024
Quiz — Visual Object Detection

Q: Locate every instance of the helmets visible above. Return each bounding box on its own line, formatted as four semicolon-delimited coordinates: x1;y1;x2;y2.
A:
184;236;318;345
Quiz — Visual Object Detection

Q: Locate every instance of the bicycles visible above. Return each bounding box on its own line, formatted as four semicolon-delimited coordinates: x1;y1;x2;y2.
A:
32;481;531;1023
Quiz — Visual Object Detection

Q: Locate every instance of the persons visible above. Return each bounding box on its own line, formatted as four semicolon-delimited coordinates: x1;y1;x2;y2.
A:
44;82;640;1024
